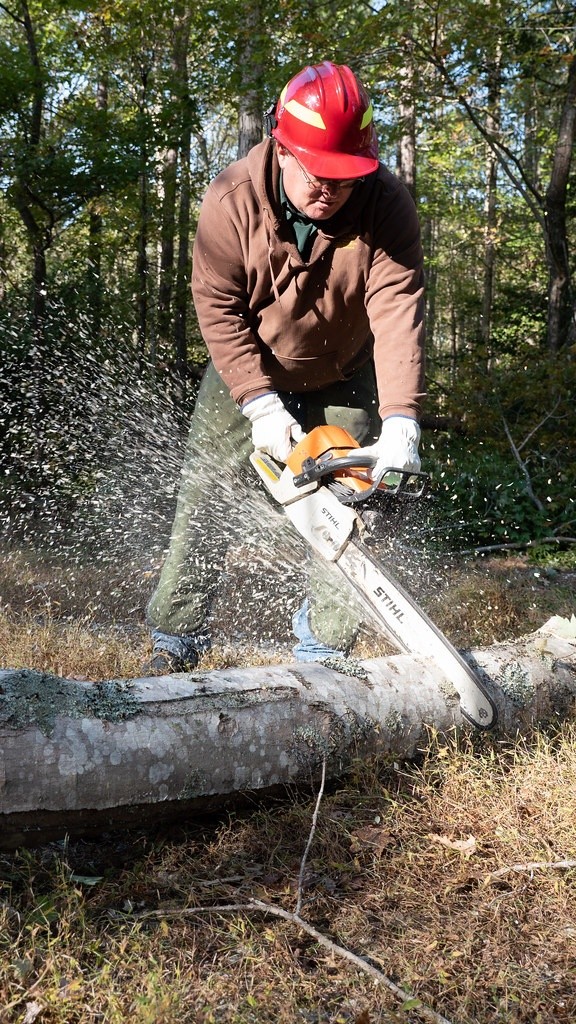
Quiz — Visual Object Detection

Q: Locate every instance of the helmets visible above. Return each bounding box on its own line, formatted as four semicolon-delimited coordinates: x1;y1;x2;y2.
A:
272;62;379;179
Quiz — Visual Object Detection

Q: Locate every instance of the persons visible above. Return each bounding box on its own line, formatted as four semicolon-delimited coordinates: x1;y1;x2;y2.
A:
140;60;428;678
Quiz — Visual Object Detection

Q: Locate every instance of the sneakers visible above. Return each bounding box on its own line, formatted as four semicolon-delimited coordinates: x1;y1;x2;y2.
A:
292;599;350;660
141;628;211;678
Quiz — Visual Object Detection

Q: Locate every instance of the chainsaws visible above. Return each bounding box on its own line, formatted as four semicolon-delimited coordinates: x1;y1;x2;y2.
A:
249;424;499;733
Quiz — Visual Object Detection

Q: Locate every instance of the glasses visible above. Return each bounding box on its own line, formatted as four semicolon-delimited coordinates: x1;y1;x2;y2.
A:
289;152;365;190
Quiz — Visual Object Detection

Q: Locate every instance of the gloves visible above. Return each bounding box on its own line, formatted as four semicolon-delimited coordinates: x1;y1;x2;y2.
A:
347;414;421;486
239;389;307;464
